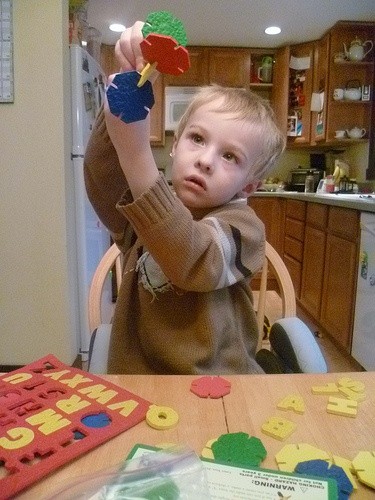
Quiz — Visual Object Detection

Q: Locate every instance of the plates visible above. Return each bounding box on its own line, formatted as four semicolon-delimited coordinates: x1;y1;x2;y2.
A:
334;136;346;139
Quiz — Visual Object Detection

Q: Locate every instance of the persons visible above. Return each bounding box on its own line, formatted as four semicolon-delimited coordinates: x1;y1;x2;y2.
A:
84;22;286;375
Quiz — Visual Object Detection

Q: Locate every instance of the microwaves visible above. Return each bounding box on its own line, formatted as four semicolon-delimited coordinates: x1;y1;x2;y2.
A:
165;86;246;133
288;168;319;186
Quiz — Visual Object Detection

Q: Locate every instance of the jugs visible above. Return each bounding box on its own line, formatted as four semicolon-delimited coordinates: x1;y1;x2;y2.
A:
258;64;272;83
343;79;362;101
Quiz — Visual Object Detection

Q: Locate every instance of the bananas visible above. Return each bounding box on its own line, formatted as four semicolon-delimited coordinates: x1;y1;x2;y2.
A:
333;163;345;184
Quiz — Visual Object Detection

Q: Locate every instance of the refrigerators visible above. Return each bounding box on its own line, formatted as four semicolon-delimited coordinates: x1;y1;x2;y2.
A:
70;44;113;365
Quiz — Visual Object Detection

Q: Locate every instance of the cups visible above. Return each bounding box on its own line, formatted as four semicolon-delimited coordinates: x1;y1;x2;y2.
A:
333;89;343;100
335;130;345;136
334;54;345;62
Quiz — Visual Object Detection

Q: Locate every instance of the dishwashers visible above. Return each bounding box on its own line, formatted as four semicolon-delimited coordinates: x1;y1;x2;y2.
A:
350;212;375;372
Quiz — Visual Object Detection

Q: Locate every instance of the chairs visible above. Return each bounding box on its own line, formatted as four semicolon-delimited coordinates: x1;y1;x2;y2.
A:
87;235;329;374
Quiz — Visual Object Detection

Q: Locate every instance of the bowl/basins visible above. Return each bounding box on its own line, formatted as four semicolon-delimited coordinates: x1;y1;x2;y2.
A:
262;184;285;191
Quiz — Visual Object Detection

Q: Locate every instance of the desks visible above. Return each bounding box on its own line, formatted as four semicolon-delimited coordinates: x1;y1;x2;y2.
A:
0;371;375;500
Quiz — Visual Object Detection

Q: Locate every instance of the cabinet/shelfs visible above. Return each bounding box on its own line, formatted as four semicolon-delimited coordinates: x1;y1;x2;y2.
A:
99;20;375;147
247;197;361;356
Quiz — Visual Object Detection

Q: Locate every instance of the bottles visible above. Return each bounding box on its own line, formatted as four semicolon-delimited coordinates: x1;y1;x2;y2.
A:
325;176;333;192
305;176;314;193
349;179;357;193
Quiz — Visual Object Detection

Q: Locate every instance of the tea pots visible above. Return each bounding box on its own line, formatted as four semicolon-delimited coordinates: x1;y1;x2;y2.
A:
344;127;366;139
342;35;373;61
